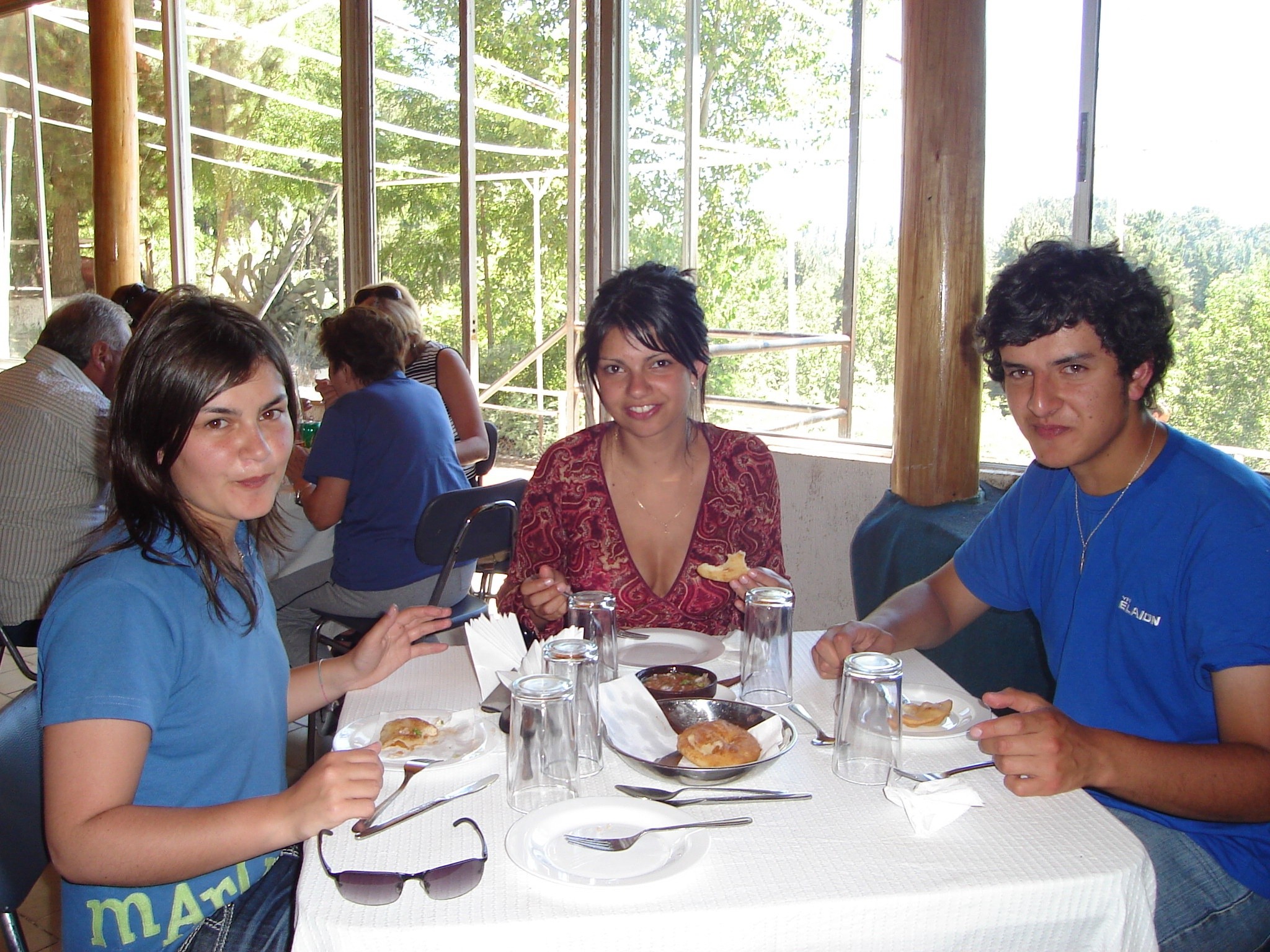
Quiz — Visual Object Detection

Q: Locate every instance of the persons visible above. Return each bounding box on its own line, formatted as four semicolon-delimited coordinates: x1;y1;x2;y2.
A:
810;240;1270;952
497;261;796;637
1147;404;1170;424
36;284;452;952
0;283;490;736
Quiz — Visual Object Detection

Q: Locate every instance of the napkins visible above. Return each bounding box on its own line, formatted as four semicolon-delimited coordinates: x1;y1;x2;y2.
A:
719;629;769;666
883;776;986;836
464;612;584;702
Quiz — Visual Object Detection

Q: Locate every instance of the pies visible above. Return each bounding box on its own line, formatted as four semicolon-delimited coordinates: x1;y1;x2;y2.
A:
677;719;761;767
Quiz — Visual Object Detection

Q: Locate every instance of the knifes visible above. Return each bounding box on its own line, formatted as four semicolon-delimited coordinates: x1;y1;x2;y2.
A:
660;793;811;808
354;774;500;840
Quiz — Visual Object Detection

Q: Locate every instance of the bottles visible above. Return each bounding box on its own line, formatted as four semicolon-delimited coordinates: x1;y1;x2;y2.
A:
292;371;304;444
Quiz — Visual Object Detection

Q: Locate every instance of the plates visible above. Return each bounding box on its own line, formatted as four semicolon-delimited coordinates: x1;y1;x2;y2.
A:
833;682;992;739
616;628;724;669
713;684;736;701
505;796;710;886
332;709;501;772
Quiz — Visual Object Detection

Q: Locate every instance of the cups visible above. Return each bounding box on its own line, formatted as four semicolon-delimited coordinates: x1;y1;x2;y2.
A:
568;591;618;684
739;586;795;707
507;674;578;814
541;639;603;780
831;652;903;785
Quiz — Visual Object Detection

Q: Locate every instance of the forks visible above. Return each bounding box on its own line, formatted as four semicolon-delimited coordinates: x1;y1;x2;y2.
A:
563;817;753;851
564;592;651;639
352;758;444;834
894;760;995;782
789;703;848;746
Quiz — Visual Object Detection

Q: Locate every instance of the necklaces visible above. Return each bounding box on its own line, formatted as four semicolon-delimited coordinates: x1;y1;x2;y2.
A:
1075;419;1157;574
615;430;695;534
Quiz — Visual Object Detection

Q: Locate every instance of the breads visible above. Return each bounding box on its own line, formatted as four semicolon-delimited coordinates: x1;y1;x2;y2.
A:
887;699;953;729
697;550;751;582
380;717;439;751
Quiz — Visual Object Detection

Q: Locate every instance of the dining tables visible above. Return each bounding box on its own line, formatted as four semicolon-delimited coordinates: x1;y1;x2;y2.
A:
258;485;342;584
290;631;1159;952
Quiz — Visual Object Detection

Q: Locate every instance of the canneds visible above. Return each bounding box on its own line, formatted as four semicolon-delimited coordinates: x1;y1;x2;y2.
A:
300;416;320;448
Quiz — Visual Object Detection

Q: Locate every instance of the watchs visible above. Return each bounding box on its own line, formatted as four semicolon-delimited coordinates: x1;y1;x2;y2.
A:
293;483;314;507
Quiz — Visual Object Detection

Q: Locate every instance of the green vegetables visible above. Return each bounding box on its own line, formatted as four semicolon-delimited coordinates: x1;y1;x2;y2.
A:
643;672;708;687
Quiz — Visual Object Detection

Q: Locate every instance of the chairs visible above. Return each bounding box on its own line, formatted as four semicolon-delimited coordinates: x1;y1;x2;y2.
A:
0;618;44;681
0;683;50;952
306;478;529;767
464;420;499;601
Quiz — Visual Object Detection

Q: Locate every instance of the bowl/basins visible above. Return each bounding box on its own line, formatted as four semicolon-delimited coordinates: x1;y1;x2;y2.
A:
600;699;798;786
636;664;717;699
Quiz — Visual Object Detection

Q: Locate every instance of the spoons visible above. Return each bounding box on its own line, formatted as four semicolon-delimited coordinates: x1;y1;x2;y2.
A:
499;704;537;780
615;785;783;800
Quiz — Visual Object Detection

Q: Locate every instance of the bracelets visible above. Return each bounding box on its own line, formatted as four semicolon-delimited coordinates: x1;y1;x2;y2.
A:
318;658;339;710
